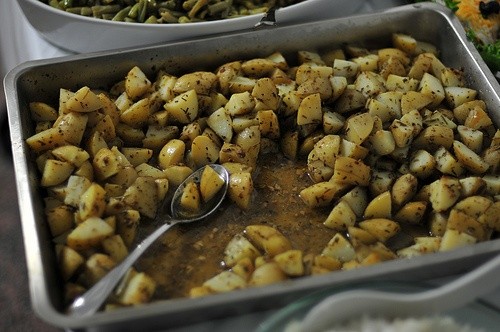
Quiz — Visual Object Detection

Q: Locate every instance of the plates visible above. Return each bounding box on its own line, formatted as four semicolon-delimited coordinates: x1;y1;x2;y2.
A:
17;0;320;54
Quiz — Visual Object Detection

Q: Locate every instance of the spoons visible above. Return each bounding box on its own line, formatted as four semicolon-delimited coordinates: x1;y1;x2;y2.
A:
69;164;229;318
